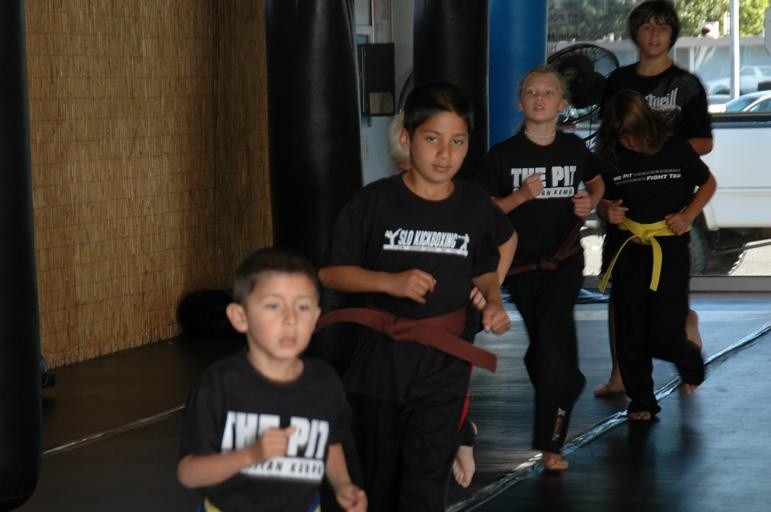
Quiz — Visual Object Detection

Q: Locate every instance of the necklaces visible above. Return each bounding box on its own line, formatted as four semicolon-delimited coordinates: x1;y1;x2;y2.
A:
522;130;560;142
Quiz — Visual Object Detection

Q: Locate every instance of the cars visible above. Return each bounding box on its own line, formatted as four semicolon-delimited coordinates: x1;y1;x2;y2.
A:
705;64;771;111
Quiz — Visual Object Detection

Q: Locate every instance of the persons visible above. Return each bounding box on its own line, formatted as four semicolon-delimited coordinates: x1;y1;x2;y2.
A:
595;0;714;401
468;64;608;472
174;243;370;512
592;88;716;425
318;79;507;512
385;101;519;489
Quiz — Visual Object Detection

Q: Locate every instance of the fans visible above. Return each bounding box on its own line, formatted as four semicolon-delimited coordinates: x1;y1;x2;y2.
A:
547;41;620;126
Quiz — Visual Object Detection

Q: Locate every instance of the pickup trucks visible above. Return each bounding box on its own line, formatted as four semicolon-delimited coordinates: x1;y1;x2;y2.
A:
557;112;771;277
